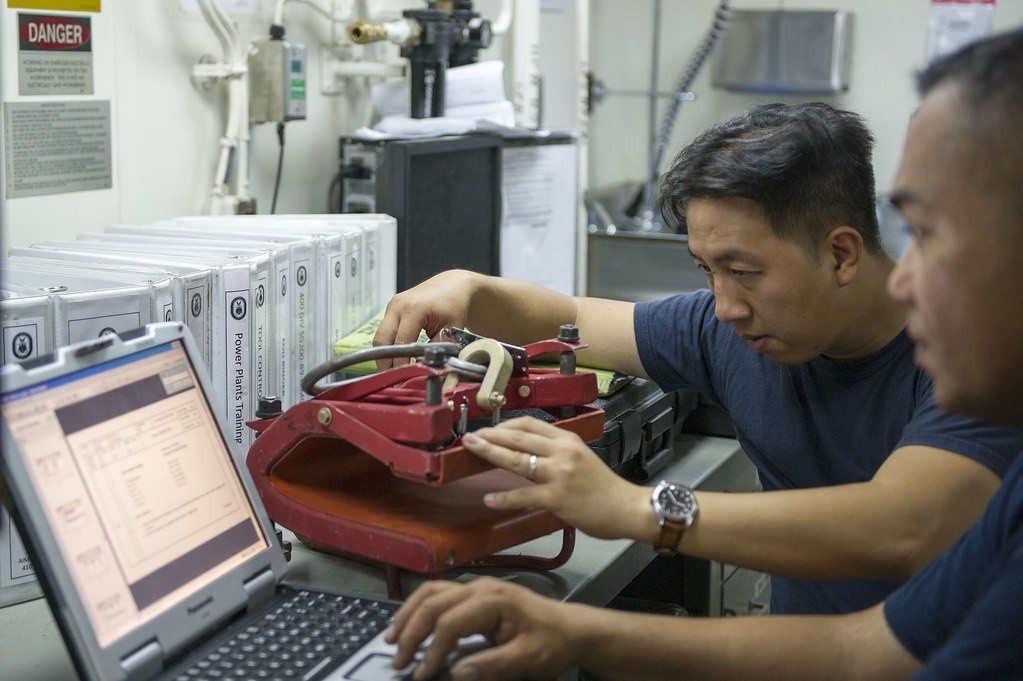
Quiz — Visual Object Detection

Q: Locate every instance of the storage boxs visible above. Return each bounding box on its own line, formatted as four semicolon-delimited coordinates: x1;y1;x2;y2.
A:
0;209;400;609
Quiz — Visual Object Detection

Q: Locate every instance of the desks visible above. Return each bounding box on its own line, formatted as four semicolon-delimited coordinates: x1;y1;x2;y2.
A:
0;437;778;681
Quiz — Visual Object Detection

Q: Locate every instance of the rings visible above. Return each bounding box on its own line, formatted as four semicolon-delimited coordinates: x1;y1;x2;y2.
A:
527;454;537;481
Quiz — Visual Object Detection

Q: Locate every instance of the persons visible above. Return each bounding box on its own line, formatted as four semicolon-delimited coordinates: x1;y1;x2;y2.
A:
374;103;1023;617
383;25;1023;681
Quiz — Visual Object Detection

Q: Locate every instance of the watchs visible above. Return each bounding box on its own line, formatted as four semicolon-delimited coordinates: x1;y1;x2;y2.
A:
651;480;698;554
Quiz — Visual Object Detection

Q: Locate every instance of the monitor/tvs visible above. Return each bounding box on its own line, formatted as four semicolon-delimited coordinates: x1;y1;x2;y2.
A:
382;130;503;303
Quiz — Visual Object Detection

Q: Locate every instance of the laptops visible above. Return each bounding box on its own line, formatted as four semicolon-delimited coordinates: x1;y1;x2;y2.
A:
0;321;496;681
